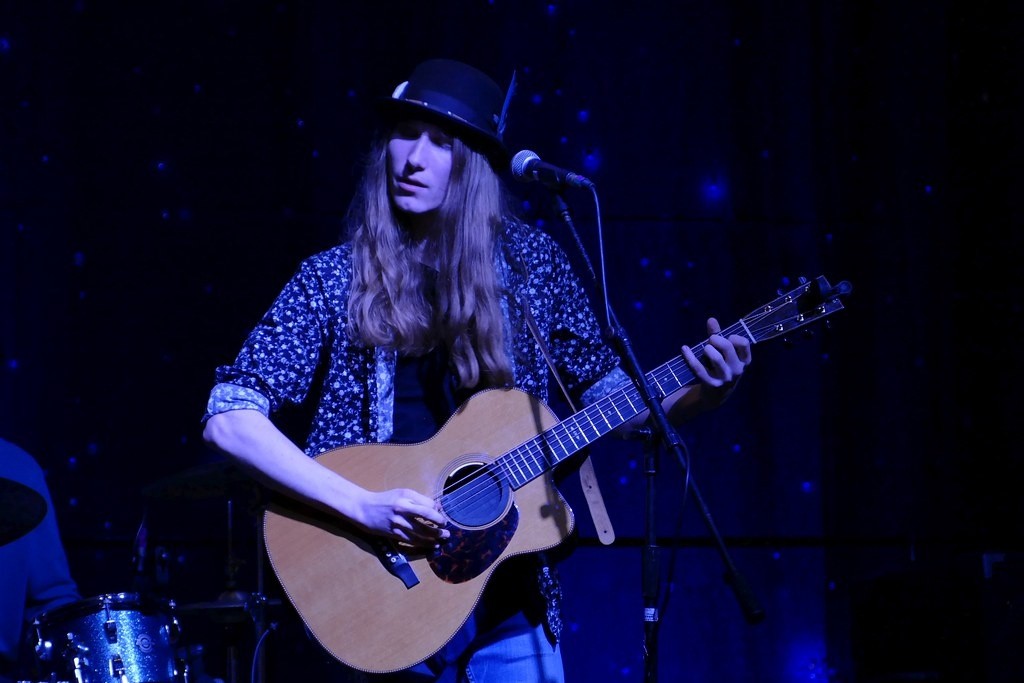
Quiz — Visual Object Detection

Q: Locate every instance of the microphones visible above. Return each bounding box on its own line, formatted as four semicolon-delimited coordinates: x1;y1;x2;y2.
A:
138;524;148;572
509;150;595;192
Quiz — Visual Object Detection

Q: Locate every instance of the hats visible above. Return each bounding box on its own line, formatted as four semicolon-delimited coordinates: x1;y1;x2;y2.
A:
378;59;509;146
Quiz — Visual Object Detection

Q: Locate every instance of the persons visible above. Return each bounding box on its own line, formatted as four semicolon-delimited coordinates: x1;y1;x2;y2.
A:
0;438;83;683
202;54;753;683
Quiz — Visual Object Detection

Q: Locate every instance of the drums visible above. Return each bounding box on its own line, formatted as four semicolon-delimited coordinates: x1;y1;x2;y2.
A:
28;589;193;683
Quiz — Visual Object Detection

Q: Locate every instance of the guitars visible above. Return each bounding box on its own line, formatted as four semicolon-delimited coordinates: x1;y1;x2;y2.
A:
256;268;868;677
